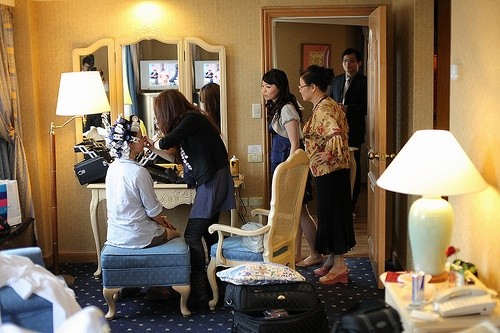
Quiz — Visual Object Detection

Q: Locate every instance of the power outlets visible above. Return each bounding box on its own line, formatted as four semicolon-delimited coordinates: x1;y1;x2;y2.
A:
248;145;261;154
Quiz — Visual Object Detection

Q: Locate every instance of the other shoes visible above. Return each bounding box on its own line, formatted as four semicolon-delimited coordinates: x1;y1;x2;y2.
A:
352;211;356;222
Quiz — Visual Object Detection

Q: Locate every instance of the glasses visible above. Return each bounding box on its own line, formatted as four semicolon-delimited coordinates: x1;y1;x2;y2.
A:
297;83;311;91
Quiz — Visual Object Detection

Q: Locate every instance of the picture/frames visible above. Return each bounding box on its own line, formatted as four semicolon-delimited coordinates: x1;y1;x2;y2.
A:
301;42;331;75
193;60;221;90
140;59;179;91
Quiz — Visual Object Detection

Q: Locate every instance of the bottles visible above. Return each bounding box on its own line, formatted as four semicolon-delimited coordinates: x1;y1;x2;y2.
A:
230;154;239;178
457;268;465;287
448;266;456;287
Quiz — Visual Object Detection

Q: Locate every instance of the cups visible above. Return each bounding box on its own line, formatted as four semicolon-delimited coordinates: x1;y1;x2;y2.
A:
411;270;426;305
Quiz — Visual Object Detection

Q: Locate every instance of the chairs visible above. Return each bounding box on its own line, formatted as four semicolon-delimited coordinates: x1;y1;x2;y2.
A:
331;301;404;333
207;148;310;310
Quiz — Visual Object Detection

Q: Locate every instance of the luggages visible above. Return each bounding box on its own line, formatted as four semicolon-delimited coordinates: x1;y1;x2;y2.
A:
224;282;329;332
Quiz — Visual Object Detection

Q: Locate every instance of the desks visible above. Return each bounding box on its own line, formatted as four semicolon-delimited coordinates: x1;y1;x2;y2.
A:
380;267;500;333
87;176;245;280
0;216;37;249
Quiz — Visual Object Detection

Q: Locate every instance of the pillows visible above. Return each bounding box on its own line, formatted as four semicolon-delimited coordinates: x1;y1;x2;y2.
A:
216;260;305;285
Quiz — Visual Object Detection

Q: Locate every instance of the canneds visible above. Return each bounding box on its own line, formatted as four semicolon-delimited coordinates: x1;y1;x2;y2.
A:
411;272;425;305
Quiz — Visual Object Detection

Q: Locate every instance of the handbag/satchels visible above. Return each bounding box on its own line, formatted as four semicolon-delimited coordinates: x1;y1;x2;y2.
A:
0;180;22;228
332;298;404;333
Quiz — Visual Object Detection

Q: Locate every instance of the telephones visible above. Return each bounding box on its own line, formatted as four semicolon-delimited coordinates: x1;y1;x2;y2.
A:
431;286;494;318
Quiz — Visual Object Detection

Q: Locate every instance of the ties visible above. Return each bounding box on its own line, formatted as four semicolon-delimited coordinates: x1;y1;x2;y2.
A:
346;76;352;85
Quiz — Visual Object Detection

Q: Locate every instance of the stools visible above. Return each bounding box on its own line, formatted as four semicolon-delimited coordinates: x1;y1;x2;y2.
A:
102;238;191;319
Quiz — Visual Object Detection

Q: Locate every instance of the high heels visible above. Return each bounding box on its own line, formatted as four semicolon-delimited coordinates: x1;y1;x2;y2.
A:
313;264;332;276
319;269;350;284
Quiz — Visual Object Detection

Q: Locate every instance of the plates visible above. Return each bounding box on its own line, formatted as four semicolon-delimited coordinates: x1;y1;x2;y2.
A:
401;283;436;303
396;273;432;283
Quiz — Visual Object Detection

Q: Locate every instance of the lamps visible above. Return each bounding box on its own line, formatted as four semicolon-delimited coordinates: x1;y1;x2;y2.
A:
376;130;488;283
48;69;111;284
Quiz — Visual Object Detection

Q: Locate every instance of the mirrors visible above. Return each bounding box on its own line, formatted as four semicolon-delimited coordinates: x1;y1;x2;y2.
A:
72;37;229;161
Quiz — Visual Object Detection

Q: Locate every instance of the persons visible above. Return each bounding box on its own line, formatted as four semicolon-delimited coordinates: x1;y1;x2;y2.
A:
326;48;366;219
140;90;234;306
261;69;323;266
298;65;356;284
106;115;180;248
200;83;221;131
80;54;109;132
